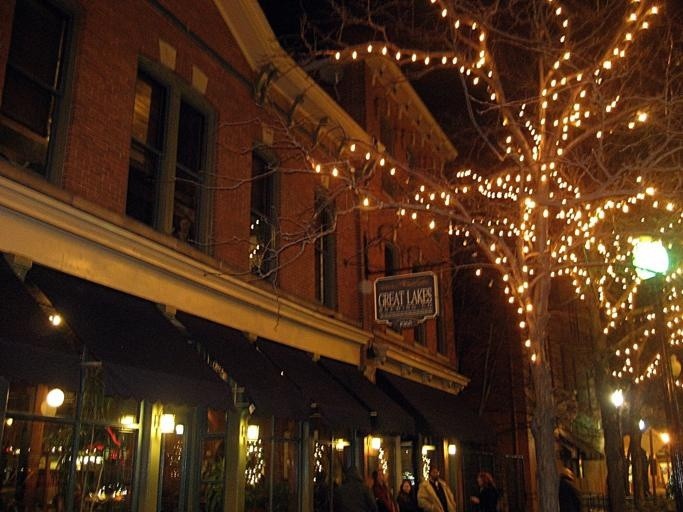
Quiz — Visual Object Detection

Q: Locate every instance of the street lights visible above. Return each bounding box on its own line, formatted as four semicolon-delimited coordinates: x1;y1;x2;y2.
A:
627;232;683;512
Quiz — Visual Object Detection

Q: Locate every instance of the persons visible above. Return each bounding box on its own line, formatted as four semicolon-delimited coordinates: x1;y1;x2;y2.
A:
395;477;422;512
414;464;458;512
311;470;335;512
557;468;584;512
370;469;399;512
332;466;379;512
467;470;500;512
